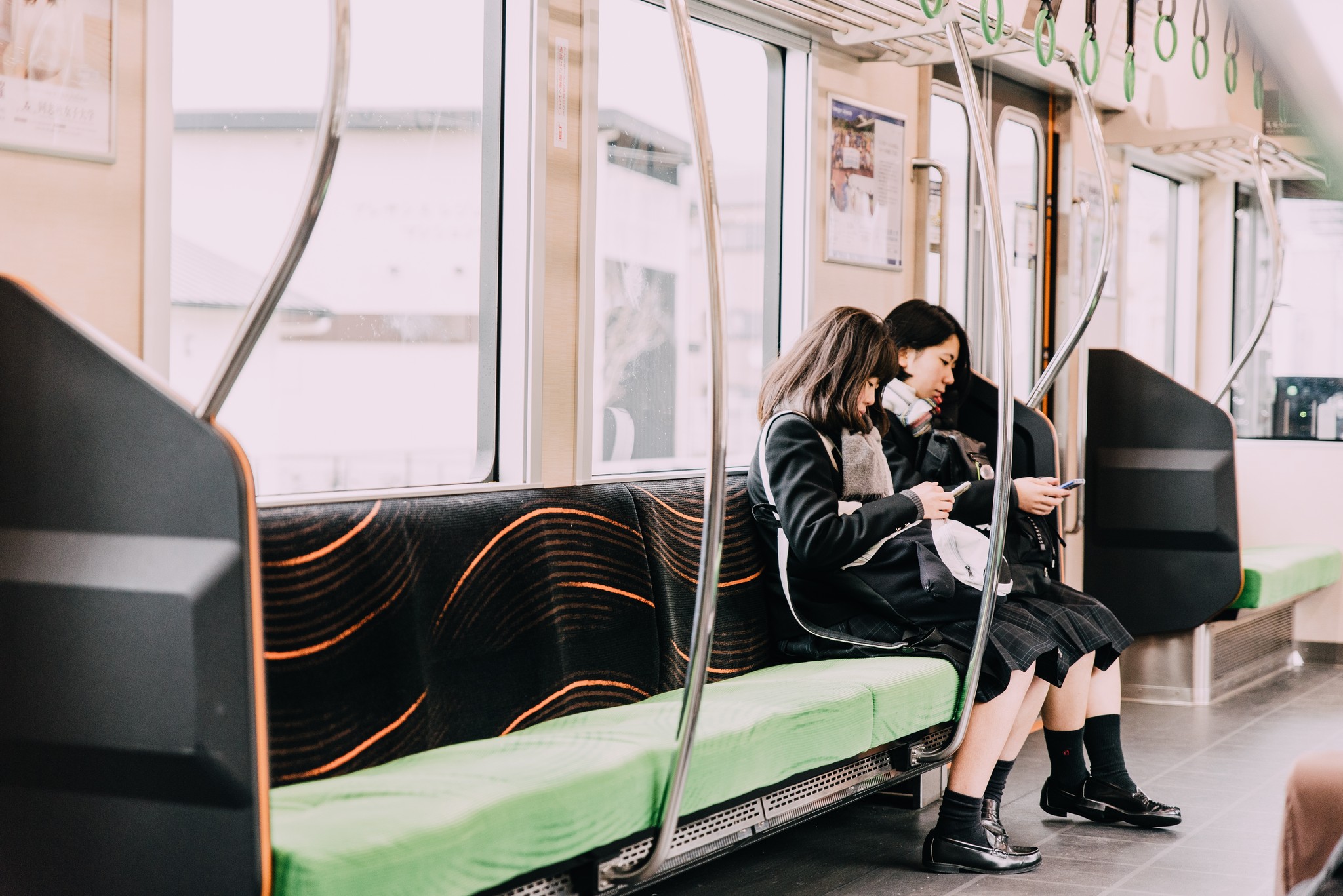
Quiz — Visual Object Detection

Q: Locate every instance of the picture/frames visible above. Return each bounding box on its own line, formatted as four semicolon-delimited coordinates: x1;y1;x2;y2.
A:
824;93;910;273
0;0;120;163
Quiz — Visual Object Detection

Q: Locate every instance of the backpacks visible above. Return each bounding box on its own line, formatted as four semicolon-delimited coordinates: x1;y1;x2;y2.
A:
913;428;1067;600
751;410;1013;681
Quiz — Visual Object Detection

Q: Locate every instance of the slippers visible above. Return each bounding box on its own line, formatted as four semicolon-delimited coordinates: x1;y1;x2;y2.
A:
1040;777;1123;824
1076;776;1182;828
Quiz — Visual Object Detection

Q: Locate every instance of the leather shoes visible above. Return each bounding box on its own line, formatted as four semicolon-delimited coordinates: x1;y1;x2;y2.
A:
919;829;1040;874
981;798;1008;844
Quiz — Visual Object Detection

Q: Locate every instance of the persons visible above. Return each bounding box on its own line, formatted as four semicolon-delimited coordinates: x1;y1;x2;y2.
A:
879;296;1186;832
749;303;1073;874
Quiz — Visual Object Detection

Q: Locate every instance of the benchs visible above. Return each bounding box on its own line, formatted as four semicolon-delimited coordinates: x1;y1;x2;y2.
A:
1119;547;1343;704
257;475;962;896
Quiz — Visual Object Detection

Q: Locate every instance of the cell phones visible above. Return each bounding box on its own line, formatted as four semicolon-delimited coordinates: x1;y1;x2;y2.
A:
951;481;972;497
1044;479;1086;498
